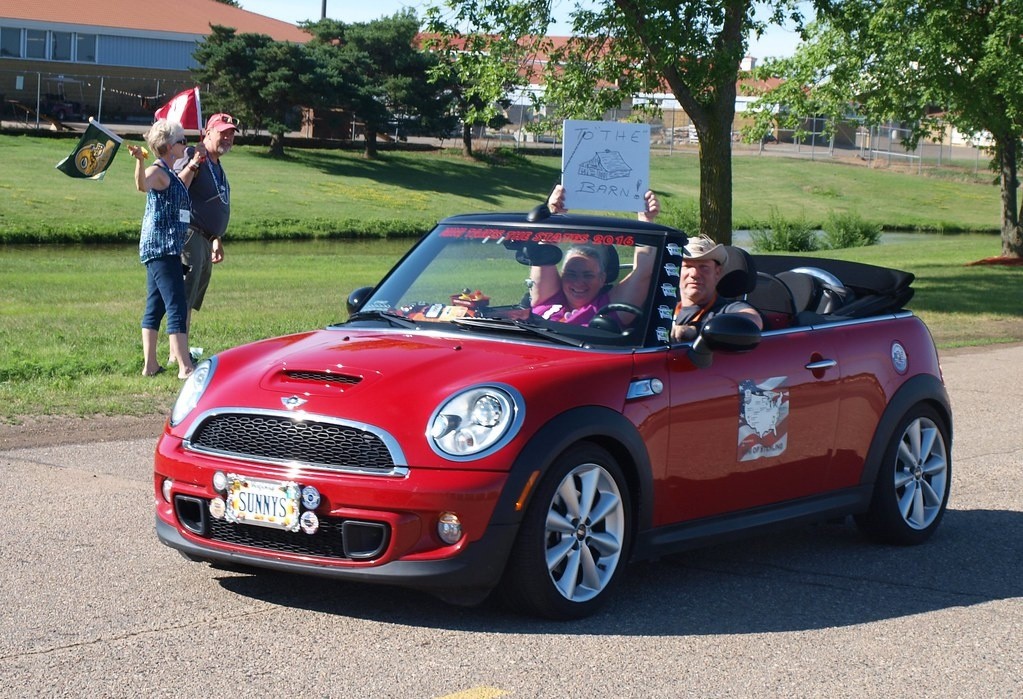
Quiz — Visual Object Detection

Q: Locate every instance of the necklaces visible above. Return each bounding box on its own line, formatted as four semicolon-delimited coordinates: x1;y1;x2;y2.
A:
205;149;229;205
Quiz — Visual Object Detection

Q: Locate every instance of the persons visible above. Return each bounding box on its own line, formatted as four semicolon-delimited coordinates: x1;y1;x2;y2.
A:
127;118;197;381
524;184;660;329
669;234;764;344
167;112;241;367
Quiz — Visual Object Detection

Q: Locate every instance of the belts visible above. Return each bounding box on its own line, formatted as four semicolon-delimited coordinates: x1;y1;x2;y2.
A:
191;224;215;241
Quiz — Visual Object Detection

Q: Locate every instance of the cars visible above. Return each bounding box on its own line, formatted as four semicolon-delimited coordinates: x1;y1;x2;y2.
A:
35;93;88;122
154;204;953;618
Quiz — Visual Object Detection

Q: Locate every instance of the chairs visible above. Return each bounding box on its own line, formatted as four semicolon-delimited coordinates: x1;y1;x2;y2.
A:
717;243;756;302
772;271;821;313
572;240;620;295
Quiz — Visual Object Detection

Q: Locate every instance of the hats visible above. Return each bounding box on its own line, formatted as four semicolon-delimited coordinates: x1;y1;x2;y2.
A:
682;237;728;269
208;114;239;134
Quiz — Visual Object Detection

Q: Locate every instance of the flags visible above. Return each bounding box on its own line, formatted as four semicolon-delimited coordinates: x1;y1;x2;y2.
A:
154;87;203;131
54;119;124;179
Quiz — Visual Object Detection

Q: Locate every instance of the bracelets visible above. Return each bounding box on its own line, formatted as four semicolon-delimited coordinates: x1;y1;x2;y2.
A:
187;159;199;172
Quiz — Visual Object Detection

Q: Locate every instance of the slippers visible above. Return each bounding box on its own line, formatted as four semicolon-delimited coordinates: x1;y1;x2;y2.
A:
147;366;167;375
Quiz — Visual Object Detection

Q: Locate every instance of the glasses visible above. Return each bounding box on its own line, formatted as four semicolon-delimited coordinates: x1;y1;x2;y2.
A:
176;139;188;146
207;115;240;127
